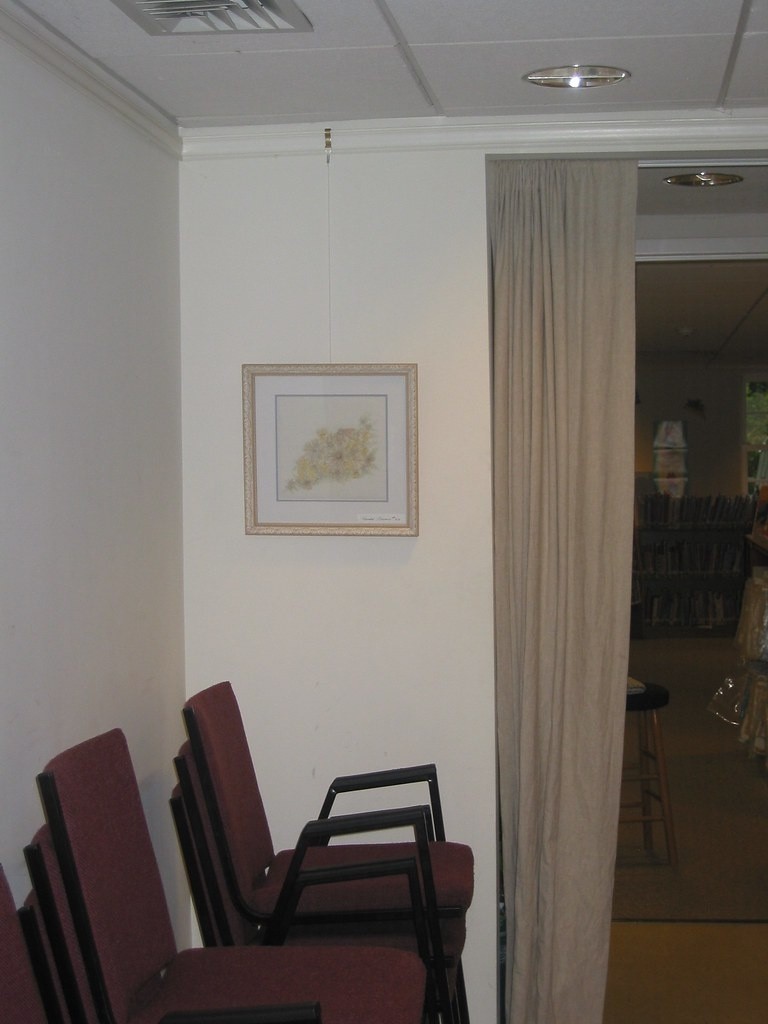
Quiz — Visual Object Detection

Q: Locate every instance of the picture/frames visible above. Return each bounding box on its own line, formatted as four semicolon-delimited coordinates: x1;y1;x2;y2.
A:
241;362;421;538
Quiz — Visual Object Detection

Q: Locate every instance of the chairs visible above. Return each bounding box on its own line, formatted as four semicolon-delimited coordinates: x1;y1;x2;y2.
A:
0;679;475;1024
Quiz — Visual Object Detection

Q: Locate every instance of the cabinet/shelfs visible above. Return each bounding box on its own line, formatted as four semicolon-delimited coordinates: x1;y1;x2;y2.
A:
635;526;750;640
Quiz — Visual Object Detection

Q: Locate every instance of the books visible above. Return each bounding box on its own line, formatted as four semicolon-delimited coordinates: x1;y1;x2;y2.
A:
631;537;747;572
644;586;743;624
642;491;756;525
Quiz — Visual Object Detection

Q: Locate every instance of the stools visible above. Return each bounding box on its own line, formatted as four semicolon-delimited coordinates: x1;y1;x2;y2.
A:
618;682;679;864
